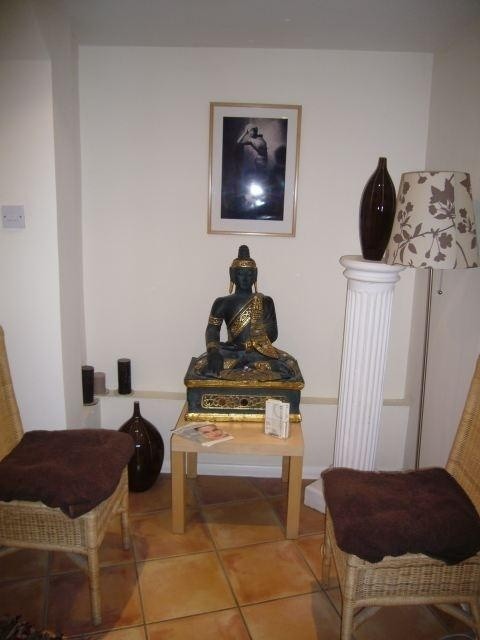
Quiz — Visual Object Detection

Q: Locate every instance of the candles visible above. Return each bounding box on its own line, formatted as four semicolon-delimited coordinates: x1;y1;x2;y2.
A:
117;359;131;395
82;366;94;403
94;373;105;394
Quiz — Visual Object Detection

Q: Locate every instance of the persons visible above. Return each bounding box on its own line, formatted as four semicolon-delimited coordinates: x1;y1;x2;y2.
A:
194;424;224;439
194;245;300;381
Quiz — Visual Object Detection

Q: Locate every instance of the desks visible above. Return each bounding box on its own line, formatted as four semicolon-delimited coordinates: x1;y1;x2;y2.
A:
170;402;303;540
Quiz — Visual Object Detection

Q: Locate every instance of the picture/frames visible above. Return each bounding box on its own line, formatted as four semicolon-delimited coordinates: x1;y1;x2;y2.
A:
207;102;302;238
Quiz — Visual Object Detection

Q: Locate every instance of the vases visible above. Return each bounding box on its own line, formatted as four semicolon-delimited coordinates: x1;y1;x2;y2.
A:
119;401;164;493
360;157;397;261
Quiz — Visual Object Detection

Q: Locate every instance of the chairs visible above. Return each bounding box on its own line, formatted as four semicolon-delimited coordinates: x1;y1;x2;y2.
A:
0;327;131;626
321;355;480;639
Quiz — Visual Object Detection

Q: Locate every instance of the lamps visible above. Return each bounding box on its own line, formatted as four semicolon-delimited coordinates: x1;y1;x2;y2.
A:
383;171;480;470
